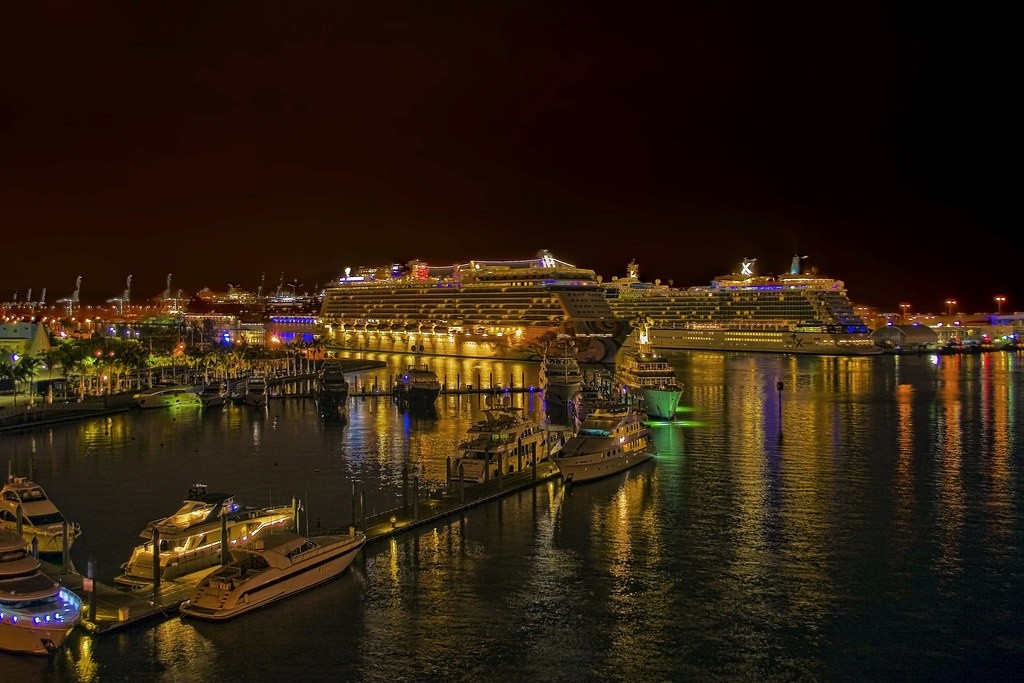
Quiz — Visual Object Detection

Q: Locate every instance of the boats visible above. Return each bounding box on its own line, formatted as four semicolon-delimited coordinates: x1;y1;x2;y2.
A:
1;460;81;552
393;337;441;414
132;382;202;408
177;529;370;621
1;524;85;661
534;332;587;397
111;482;304;590
607;305;689;420
311;351;350;411
200;368;270;408
544;369;659;484
444;382;567;484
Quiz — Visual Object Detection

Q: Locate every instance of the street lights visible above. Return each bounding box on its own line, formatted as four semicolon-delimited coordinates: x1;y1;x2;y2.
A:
995;296;1006;316
947;298;958;317
900;304;911;321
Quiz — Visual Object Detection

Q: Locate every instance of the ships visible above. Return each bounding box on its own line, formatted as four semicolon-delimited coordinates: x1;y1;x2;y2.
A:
597;256;889;358
311;255;637;367
